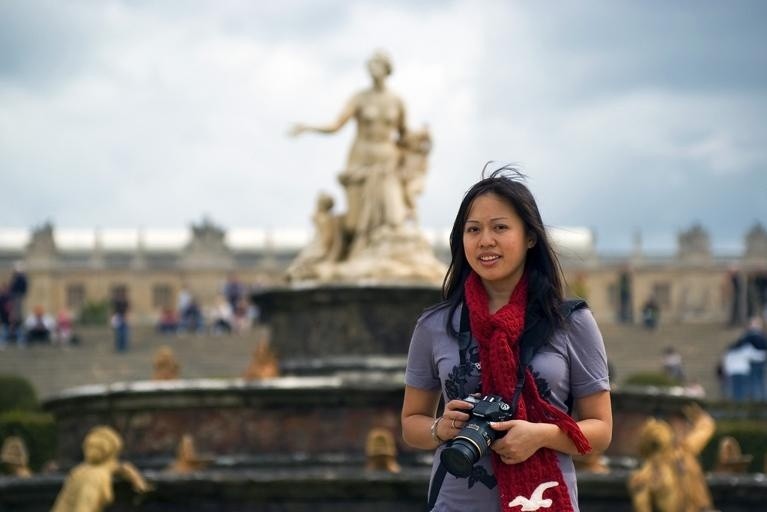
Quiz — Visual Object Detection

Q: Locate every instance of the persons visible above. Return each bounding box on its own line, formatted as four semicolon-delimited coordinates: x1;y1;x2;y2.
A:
365;427;399;473
151;270;263;337
401;157;614;512
622;288;766;512
153;345;179;382
288;42;436;265
247;341;275;380
0;272;78;348
110;284;131;353
574;453;610;475
283;192;336;274
1;437;30;477
55;427;147;511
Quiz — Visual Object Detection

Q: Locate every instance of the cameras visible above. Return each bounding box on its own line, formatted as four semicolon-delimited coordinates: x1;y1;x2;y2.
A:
440;394;514;478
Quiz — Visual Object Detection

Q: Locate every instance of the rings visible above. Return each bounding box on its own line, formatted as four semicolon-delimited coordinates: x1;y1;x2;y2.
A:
452;420;457;428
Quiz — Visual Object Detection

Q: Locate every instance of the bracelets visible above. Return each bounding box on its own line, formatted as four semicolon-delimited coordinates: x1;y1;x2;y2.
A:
430;416;447;445
